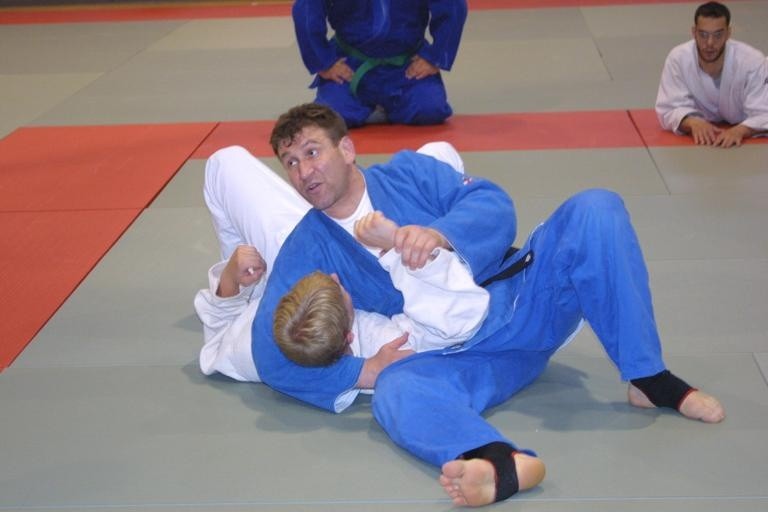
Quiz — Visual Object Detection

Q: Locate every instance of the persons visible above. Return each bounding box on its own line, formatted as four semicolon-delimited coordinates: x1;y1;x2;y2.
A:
190;140;492;395
288;0;469;129
654;0;768;147
249;102;725;508
